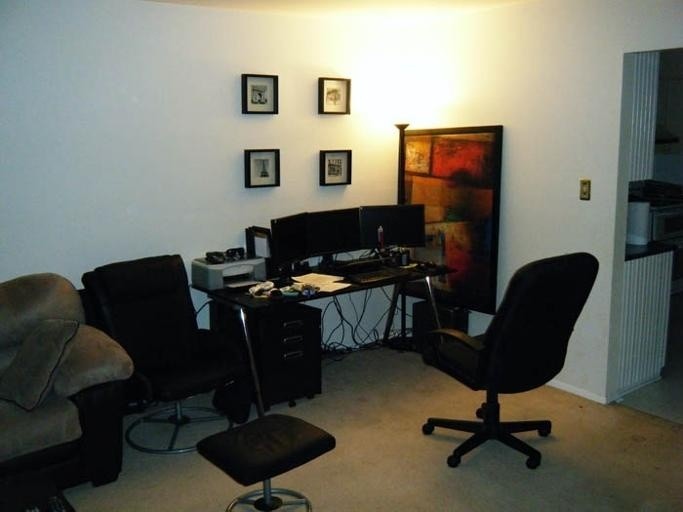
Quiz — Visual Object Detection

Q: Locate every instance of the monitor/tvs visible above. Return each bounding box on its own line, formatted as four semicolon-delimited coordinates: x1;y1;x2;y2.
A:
306;207;361;269
270;212;307;272
359;203;427;258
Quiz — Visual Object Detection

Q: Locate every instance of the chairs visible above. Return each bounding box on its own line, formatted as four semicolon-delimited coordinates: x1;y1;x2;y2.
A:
422;252;599;470
80;253;233;455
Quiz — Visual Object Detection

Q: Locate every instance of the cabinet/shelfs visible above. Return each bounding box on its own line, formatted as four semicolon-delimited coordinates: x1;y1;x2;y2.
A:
615;242;677;401
187;259;456;405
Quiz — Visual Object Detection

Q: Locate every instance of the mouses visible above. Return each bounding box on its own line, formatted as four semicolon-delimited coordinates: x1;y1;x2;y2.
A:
416;260;438;274
206;251;225;263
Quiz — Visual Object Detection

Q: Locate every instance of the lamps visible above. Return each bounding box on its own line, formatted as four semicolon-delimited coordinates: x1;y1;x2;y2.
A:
382;119;421;353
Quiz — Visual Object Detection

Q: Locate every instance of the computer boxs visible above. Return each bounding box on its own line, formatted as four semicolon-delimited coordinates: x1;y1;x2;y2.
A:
412;301;469;344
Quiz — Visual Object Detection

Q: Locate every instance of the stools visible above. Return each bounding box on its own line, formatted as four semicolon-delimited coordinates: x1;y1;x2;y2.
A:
196;413;337;512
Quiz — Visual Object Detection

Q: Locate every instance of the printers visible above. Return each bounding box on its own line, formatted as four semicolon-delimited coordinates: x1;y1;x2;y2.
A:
192;249;267;291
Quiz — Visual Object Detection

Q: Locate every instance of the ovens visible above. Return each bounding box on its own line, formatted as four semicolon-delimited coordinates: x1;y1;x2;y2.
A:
649;205;683;296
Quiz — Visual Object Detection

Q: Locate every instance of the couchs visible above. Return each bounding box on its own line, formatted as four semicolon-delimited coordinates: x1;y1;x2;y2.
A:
0;272;136;512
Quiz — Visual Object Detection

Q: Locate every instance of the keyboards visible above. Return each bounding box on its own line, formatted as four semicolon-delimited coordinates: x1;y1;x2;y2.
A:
345;264;411;288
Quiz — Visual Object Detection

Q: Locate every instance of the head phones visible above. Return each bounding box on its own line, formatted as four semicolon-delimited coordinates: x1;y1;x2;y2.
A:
226;247;244;256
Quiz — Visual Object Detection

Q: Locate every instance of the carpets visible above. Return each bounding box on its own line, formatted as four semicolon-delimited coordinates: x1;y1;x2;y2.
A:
19;495;74;512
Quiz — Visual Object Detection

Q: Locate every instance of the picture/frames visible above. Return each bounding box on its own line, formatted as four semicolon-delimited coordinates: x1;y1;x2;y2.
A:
320;150;352;187
244;149;280;189
319;77;351;115
241;73;278;114
399;124;503;316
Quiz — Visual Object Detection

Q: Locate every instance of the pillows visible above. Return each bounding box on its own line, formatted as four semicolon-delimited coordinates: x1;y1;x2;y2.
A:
0;318;80;412
